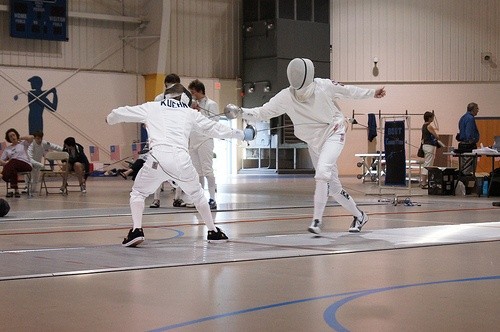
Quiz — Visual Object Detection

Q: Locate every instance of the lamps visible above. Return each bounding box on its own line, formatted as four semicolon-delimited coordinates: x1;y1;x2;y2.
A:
243;25;254;32
267;20;275;30
263;85;271;93
247;85;255;93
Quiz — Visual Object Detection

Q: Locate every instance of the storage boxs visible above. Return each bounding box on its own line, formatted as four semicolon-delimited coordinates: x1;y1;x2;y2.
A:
428;168;455;195
434;135;453;167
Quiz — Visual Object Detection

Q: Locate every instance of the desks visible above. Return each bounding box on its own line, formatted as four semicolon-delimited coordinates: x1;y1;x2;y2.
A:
356;154;385;184
442;152;500;198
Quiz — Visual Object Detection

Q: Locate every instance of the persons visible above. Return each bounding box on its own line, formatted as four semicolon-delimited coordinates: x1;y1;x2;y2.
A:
421;111;439;189
224;55;386;234
21;131;66;193
150;73;182;207
105;83;255;245
0;128;33;197
458;102;480;176
60;136;90;192
186;79;220;209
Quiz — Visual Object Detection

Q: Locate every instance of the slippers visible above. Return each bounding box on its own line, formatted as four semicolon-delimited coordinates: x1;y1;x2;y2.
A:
119;171;128;179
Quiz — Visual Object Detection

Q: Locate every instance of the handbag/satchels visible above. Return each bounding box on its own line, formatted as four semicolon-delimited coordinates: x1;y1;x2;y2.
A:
417;142;425;158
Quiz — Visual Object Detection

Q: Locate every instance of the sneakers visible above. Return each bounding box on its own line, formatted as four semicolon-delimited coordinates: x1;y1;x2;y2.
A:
121;228;145;247
307;219;322;234
349;210;369;232
207;227;229;243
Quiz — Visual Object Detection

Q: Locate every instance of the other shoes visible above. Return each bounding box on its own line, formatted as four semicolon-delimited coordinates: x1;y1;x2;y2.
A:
186;203;196;208
150;199;160;208
421;184;428;189
15;191;20;198
173;199;186;207
6;191;13;197
81;186;87;193
208;199;217;209
21;186;28;194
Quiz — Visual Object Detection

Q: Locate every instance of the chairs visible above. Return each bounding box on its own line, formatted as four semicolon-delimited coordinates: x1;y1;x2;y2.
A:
6;169;33;197
38;152;88;196
